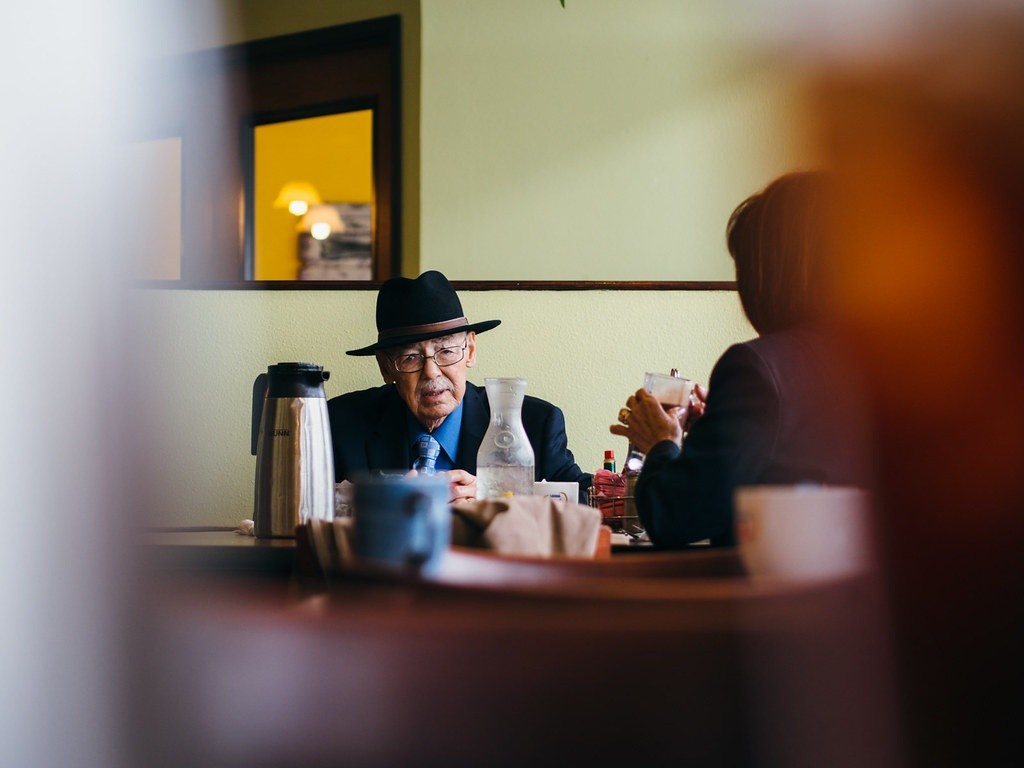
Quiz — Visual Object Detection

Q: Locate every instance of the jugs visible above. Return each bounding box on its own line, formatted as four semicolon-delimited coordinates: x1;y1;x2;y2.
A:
249;363;336;538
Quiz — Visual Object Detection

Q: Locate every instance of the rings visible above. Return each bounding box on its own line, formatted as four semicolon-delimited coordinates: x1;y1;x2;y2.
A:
619;409;630;424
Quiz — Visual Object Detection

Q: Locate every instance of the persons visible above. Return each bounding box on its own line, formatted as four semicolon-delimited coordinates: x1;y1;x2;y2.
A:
610;166;829;555
327;270;596;508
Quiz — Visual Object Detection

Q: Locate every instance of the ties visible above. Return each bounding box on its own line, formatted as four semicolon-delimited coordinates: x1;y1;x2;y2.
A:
412;435;441;477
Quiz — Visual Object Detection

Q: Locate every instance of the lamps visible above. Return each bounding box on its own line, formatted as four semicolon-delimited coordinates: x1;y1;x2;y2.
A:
272;181;346;242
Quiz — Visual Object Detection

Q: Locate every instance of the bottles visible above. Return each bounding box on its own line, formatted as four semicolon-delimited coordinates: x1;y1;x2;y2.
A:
604;451;615;475
476;378;534;501
621;438;643;475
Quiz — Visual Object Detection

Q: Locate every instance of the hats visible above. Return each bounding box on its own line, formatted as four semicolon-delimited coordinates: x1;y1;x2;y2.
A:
345;270;501;357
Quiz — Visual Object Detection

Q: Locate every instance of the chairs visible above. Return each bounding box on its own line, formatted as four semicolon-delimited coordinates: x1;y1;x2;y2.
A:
348;534;891;757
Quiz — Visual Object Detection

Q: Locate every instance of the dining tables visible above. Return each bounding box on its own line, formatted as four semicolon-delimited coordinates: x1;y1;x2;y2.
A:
139;502;879;762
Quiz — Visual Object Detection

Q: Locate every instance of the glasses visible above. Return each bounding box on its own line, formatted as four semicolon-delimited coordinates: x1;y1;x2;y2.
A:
385;338;468;373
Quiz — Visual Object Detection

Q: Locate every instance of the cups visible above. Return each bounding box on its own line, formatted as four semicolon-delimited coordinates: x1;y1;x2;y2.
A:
536;482;579;506
625;372;693;425
